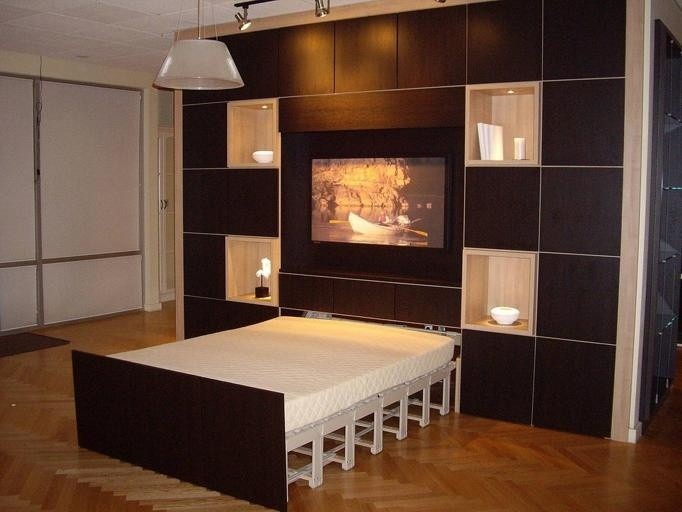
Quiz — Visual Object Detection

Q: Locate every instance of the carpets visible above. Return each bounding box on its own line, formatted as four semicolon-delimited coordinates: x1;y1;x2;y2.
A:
2;319;69;363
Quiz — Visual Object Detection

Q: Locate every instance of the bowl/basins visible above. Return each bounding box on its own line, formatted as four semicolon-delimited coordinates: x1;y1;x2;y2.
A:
491;307;520;324
251;150;273;163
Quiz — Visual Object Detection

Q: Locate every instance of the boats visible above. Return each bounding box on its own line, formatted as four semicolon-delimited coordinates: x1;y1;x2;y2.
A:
347;213;413;235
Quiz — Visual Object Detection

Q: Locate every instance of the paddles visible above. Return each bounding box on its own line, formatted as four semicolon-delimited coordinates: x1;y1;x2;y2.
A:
329;220;381;225
391;225;428;237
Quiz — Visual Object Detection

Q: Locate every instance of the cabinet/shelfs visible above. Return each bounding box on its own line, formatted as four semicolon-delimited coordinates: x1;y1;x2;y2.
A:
225;236;280;308
463;82;539;166
226;97;279;170
462;248;536;337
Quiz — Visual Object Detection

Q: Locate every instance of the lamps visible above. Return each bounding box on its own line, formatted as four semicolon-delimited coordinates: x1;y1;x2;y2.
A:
152;0;243;95
232;0;331;35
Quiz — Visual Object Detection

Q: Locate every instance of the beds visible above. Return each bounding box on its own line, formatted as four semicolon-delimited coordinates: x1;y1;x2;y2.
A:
71;262;460;512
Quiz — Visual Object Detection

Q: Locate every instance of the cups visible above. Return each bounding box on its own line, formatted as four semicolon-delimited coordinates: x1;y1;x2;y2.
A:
513;137;525;159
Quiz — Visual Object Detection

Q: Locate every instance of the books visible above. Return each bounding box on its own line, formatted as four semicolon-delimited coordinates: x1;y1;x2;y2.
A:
477;123;503;161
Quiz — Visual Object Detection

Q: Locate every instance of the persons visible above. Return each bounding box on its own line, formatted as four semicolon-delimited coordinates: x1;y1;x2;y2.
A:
358;203;365;217
379;202;387;223
